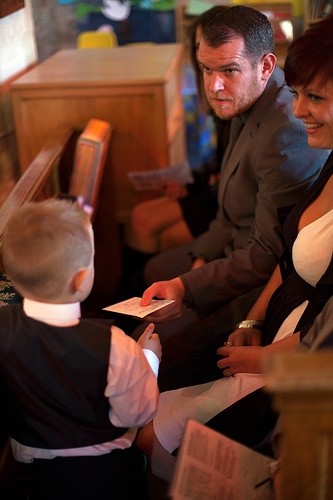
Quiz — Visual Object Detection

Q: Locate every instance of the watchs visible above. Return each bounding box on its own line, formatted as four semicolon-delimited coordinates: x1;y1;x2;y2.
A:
235;320;264;329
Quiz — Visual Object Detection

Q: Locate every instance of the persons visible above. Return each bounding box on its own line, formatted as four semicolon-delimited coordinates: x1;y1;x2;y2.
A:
116;4;333;500
0;196;162;500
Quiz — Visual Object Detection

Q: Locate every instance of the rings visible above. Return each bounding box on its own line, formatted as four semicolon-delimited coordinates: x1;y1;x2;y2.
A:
223;341;233;346
223;369;232;377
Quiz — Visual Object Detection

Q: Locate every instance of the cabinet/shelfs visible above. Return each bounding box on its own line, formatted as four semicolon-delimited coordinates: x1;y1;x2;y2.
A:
7;41;186;226
